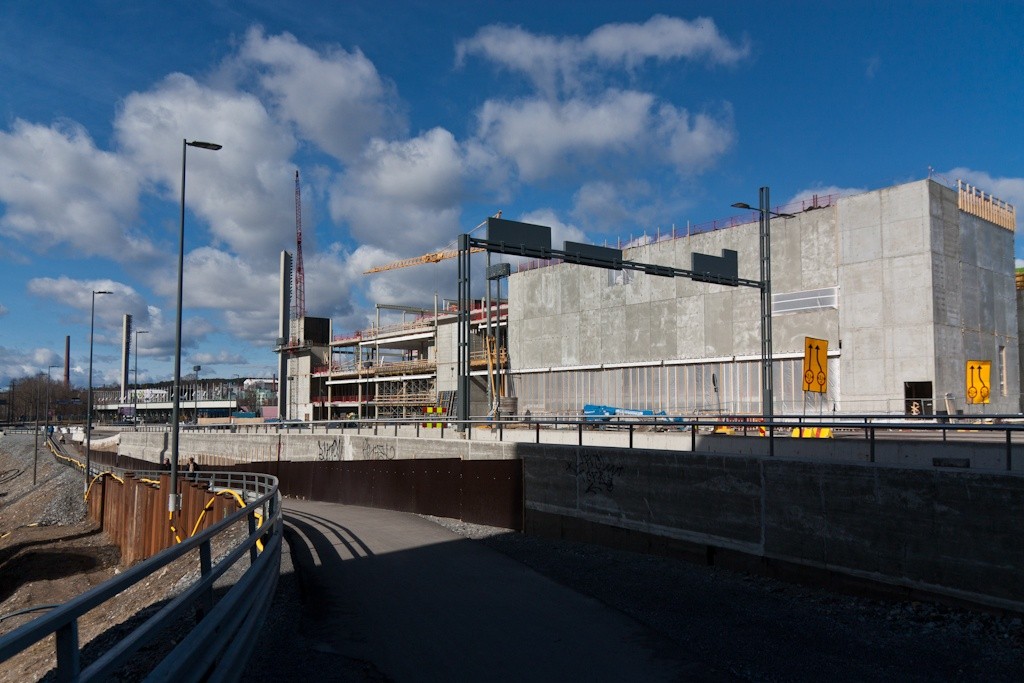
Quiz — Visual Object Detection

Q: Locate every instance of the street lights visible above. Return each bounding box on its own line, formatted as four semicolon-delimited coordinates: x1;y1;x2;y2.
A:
729;185;798;422
167;136;223;515
232;374;239;413
82;290;116;491
132;330;150;432
193;365;201;425
43;366;61;446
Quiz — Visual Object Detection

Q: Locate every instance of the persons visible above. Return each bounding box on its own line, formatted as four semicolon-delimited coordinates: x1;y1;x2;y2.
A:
59;434;66;444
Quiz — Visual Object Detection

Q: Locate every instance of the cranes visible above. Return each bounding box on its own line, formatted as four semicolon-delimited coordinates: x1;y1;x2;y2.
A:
290;168;309;319
362;209;502;277
